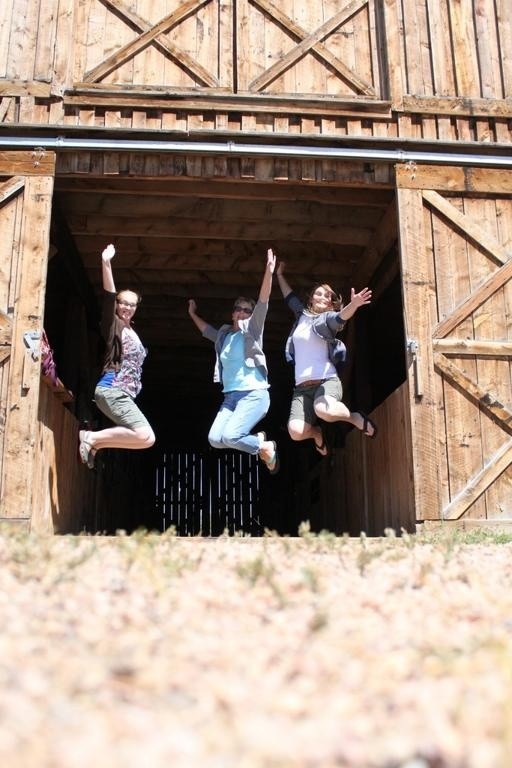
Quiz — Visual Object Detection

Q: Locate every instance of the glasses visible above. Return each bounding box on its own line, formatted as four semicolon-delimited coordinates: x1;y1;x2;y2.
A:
119;301;136;307
235;305;253;314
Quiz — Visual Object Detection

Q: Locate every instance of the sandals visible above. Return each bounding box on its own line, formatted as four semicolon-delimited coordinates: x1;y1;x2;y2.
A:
87;445;98;469
79;430;90;464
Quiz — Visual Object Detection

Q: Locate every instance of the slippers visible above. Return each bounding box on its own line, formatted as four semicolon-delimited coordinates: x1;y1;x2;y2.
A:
357;411;377;439
264;440;280;474
314;438;328;456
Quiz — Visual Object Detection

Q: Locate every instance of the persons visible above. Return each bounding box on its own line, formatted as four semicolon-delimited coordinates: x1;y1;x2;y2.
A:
189;247;281;475
276;261;377;456
79;243;156;469
